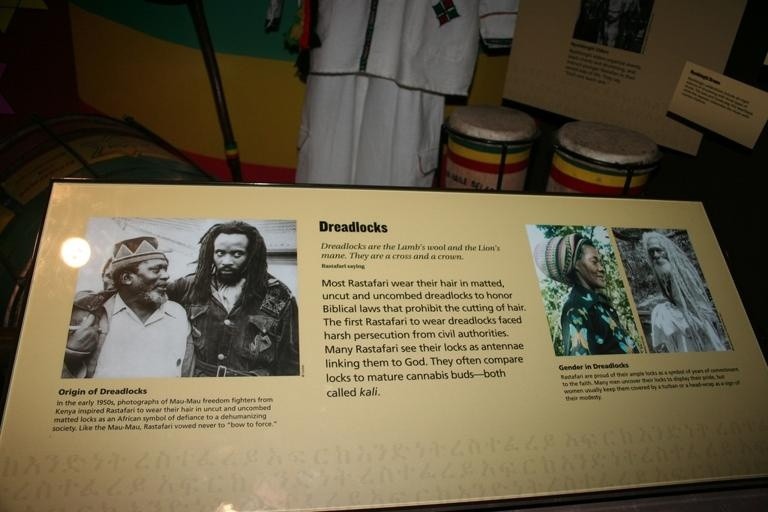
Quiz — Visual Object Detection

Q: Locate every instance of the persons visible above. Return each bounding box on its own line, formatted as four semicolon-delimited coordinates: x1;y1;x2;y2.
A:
69;220;300;378
533;233;640;357
642;231;727;353
67;236;195;379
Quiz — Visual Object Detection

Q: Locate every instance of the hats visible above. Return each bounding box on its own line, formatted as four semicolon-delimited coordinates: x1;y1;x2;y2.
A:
107;236;167;274
534;232;584;287
